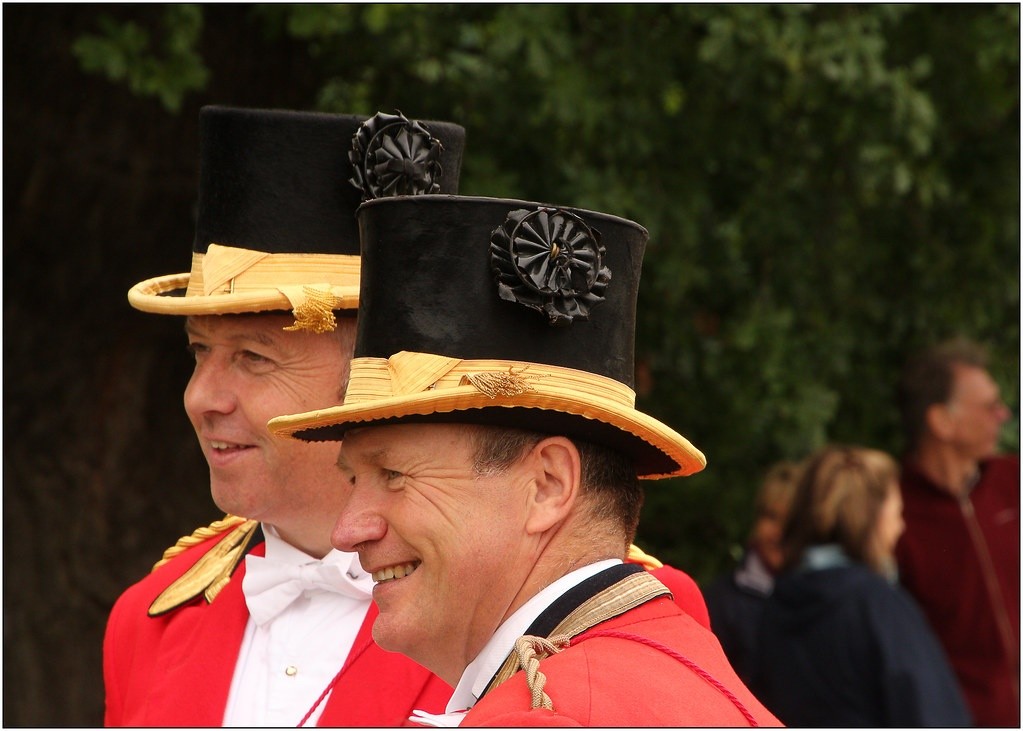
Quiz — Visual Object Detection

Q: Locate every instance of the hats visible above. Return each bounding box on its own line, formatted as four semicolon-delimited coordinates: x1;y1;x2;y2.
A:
128;108;464;330
266;193;707;481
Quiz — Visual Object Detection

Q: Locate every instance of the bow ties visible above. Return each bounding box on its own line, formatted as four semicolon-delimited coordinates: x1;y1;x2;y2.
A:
242;549;381;626
408;709;470;728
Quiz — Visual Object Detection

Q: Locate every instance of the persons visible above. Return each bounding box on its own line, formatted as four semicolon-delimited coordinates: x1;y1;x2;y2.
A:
269;195;796;728
892;329;1021;728
101;104;707;728
708;443;979;728
